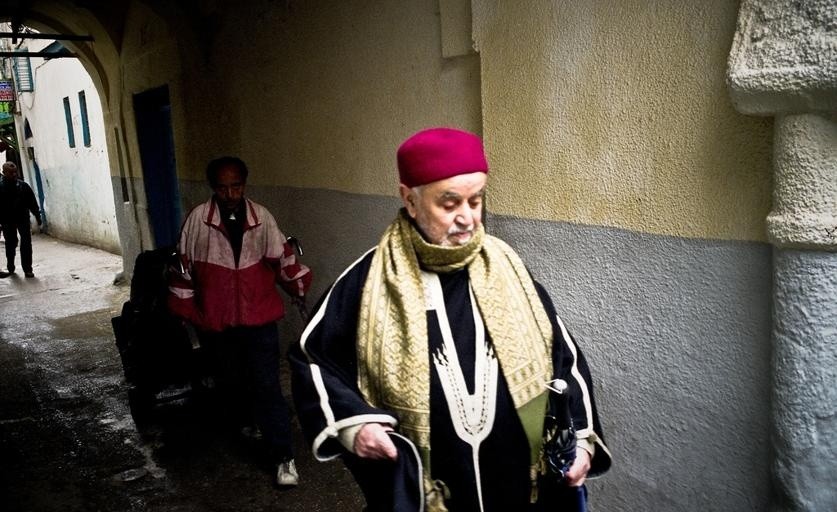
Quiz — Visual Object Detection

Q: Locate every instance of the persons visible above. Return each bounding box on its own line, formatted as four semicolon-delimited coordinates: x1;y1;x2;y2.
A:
287;126;616;511
166;155;312;489
0;161;43;279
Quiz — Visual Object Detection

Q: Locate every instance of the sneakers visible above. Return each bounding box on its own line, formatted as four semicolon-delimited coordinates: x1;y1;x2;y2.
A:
276;458;300;486
1;265;35;279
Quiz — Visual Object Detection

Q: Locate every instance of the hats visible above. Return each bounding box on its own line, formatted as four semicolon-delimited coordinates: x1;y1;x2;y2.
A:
398;124;488;185
0;160;19;179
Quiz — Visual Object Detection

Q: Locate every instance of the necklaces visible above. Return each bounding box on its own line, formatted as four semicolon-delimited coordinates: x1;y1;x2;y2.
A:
227;213;236;220
